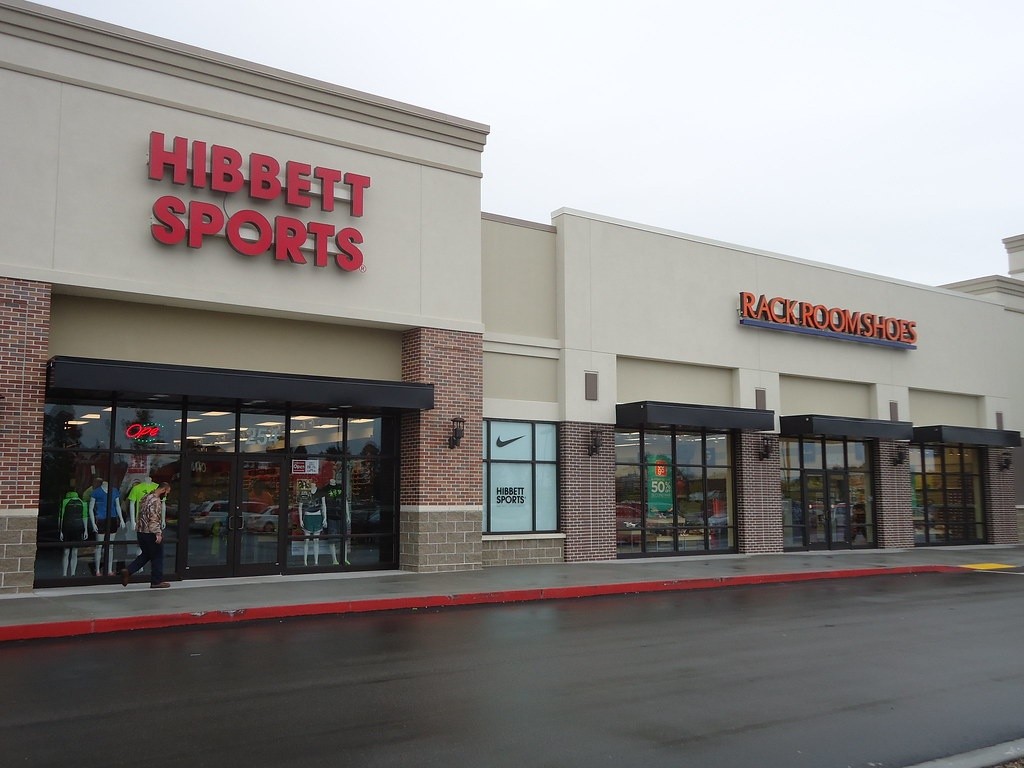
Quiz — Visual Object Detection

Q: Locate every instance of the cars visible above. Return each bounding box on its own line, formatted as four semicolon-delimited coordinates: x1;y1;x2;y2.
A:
615;505;648;544
186;500;291;535
834;503;854;527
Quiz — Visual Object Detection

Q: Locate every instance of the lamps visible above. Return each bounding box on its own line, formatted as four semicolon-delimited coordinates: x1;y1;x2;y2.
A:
588;428;607;457
448;418;466;449
957;449;960;456
1000;450;1014;472
892;445;909;466
964;449;969;457
948;448;954;456
758;436;776;461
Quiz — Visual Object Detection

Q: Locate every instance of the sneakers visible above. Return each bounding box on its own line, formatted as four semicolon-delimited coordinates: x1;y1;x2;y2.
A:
122;569;129;587
150;583;170;588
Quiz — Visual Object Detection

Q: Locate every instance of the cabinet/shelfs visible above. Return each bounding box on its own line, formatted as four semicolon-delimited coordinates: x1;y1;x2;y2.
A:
351;460;370;496
244;479;297;505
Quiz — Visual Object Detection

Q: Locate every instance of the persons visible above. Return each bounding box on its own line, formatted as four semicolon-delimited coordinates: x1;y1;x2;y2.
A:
128;477;167;574
119;482;171;588
58;492;88;577
318;479;351;565
90;481;125;577
298;483;327;566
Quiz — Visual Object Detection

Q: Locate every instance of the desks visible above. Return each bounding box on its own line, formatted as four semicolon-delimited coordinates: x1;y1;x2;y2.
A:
913;516;952;543
619;530;711;552
